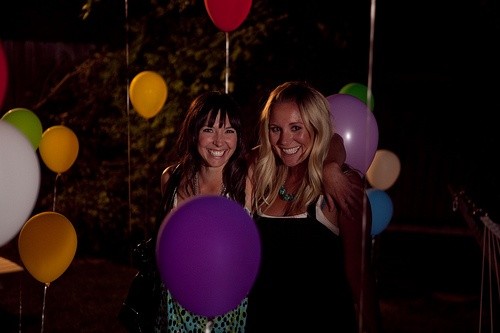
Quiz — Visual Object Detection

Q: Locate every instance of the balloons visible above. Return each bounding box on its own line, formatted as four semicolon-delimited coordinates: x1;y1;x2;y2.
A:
0;42;9;107
129;70;168;121
339;82;375;112
0;121;41;246
0;107;43;153
326;93;379;179
39;125;80;175
155;194;261;319
364;188;393;238
365;150;401;192
18;211;78;289
203;0;253;33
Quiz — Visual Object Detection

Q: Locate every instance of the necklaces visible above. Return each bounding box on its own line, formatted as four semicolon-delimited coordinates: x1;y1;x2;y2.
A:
193;169;227;197
279;185;300;202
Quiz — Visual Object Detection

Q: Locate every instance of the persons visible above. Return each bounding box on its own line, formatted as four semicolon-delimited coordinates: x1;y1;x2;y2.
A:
154;91;367;333
243;79;386;333
